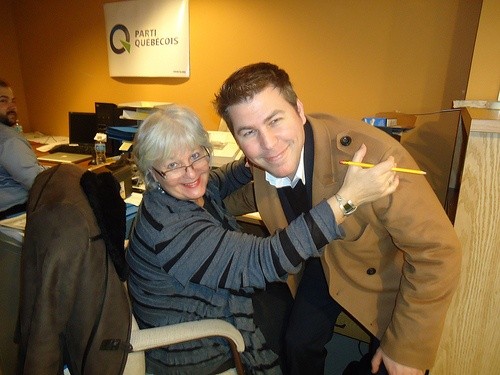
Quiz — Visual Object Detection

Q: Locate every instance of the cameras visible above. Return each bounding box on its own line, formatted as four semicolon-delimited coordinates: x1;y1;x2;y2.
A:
68;111;96;146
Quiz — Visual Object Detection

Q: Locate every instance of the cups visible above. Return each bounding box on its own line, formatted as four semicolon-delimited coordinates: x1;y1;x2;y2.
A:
95;140;106;165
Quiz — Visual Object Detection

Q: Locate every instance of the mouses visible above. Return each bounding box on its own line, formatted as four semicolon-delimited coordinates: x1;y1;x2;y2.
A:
88;159;96;165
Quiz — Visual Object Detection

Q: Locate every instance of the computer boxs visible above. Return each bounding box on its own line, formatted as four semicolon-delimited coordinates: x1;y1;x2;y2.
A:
95;102;137;157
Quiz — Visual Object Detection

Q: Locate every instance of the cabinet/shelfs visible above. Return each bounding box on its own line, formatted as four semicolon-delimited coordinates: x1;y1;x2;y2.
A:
427;107;500;375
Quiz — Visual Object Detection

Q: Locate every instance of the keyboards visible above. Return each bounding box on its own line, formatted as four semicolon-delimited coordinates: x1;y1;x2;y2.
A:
49;144;96;155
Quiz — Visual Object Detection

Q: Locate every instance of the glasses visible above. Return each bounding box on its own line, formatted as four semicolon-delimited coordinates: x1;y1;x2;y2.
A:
152;146;210;180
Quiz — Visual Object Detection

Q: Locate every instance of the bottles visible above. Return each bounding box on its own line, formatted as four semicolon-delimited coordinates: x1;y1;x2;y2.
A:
14;120;20;134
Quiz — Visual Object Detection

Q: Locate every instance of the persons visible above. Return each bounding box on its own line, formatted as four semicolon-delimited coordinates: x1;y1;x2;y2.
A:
212;62;462;375
125;104;400;375
0;80;50;220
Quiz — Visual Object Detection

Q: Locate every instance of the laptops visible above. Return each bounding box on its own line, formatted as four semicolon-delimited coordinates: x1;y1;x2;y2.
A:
37;151;93;165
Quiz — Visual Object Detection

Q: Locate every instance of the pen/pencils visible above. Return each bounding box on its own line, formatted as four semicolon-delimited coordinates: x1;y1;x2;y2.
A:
338;160;427;176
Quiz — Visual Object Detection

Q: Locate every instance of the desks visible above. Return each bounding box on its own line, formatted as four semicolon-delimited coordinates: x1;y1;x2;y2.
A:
28;139;132;200
235;212;370;343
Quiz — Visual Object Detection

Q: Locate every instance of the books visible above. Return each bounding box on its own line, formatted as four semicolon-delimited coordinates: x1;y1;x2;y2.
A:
207;130;246;167
0;213;27;243
117;100;173;120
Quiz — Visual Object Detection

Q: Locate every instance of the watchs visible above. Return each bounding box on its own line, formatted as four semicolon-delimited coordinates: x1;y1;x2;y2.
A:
335;194;358;216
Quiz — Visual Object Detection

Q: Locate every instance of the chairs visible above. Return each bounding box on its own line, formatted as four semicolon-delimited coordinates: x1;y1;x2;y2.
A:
13;163;245;375
